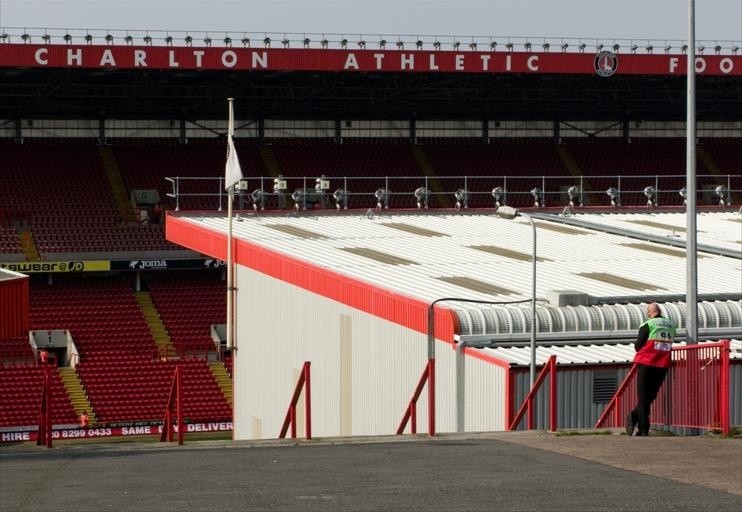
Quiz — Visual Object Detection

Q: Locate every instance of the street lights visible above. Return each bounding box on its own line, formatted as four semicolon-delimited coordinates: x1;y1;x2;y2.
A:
496;205;537;393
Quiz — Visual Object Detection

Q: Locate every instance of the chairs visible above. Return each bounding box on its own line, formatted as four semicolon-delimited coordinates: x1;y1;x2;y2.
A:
3;140;740;255
1;265;235;426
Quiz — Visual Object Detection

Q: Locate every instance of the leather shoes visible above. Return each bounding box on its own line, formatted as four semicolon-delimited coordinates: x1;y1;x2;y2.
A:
626;412;650;436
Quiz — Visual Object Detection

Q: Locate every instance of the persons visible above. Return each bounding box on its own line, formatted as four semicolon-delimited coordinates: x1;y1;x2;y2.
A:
80;410;89;428
155;201;163;224
40;347;48;363
626;303;676;436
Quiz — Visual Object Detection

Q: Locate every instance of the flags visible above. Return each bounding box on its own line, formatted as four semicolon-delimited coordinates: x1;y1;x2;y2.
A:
224;101;244;201
